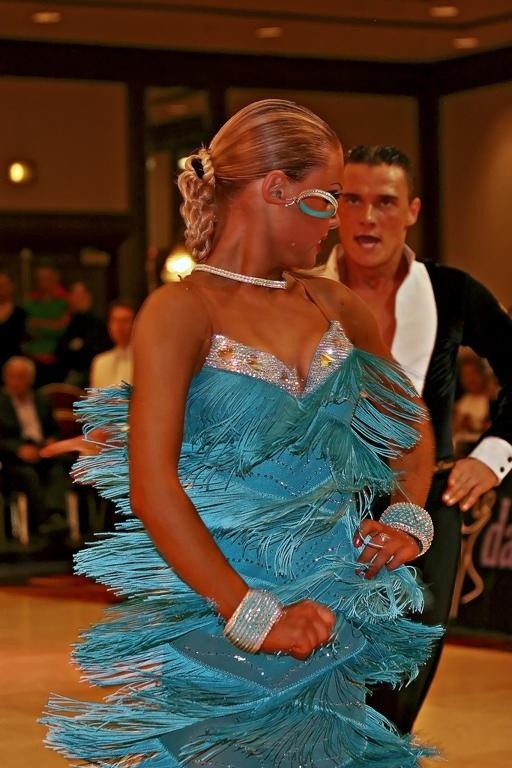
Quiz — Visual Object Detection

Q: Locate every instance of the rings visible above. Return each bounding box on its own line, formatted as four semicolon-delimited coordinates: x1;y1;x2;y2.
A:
378;532;391;542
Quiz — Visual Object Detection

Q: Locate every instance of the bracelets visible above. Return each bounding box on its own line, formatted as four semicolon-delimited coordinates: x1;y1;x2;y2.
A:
222;587;285;656
379;500;436;557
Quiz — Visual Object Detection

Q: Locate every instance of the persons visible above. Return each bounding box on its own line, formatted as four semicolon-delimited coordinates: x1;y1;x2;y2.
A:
311;142;512;737
128;97;436;768
0;262;136;542
453;350;495;435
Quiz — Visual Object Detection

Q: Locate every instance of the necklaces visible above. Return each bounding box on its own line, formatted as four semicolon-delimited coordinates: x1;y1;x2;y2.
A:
184;261;287;290
